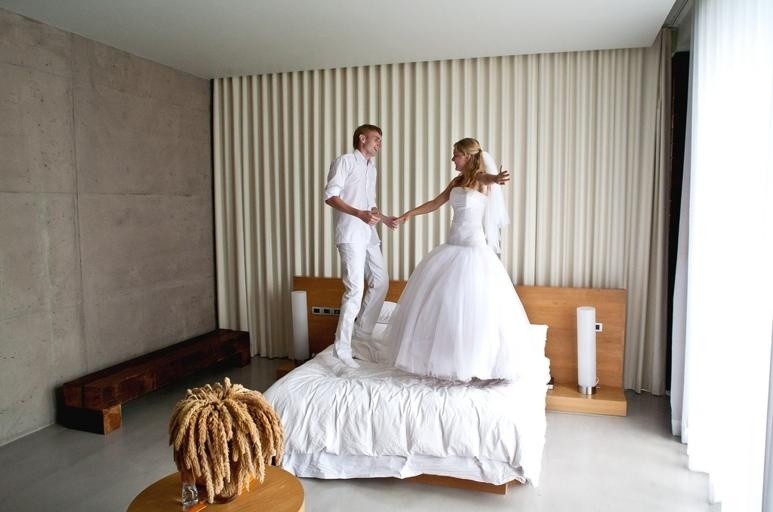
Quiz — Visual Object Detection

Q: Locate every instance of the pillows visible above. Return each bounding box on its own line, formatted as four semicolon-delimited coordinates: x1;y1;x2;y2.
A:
372;300;396;344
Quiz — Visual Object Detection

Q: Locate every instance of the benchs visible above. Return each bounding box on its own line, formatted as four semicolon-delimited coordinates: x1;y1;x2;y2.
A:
62;328;251;435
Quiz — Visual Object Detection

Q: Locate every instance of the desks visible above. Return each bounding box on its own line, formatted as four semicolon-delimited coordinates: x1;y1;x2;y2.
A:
125;462;306;512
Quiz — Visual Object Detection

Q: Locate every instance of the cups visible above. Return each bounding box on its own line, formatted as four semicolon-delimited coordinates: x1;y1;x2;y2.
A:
182;484;199;506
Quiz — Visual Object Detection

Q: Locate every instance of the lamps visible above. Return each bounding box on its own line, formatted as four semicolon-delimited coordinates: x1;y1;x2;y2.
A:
290;290;313;368
576;305;598;395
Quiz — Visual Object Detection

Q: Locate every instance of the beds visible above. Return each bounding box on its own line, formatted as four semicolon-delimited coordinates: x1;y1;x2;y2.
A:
260;340;551;497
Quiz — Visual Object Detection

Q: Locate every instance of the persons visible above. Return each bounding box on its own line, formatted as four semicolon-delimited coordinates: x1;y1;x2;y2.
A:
322;123;397;369
375;138;532;384
